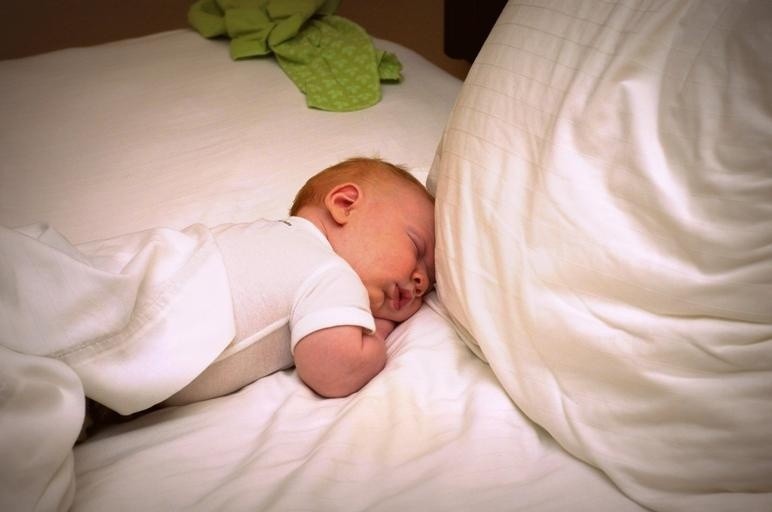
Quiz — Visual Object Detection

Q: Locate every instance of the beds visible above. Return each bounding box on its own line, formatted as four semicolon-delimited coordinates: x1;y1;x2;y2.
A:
0;23;646;512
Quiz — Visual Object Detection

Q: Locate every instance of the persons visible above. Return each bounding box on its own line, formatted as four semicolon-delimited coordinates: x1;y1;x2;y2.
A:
0;158;436;408
